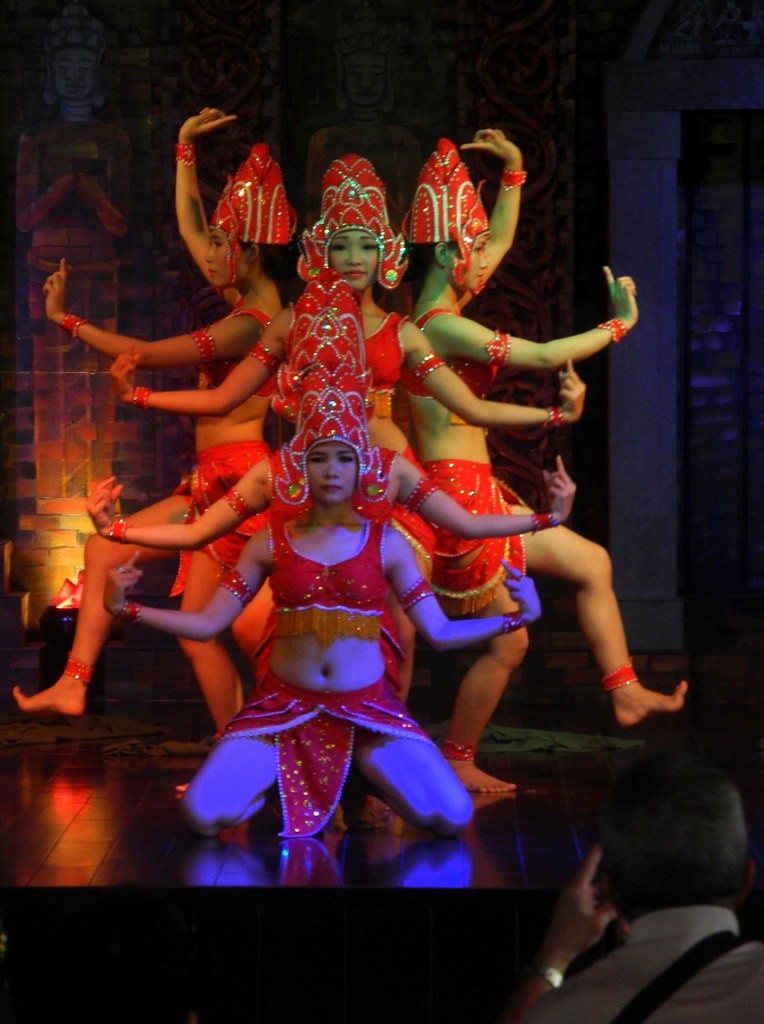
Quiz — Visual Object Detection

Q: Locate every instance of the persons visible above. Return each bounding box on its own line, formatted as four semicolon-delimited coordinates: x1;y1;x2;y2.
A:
510;748;764;1024
83;359;575;689
405;128;687;794
113;223;587;705
180;836;472;890
103;439;542;831
14;108;298;739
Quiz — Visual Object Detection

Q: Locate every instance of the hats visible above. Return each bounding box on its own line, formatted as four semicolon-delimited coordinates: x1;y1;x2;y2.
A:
399;135;494;296
206;142;300;287
269;266;395;505
295;153;409;291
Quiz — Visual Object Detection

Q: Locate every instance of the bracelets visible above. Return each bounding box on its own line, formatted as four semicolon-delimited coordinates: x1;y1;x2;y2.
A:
597;319;628;347
499;168;527;190
175;143;196;166
545;405;564;429
538;965;563;987
530;511;558;536
62;314;85;338
108;512;130;545
132;386;152;411
501;611;523;635
120;600;142;625
